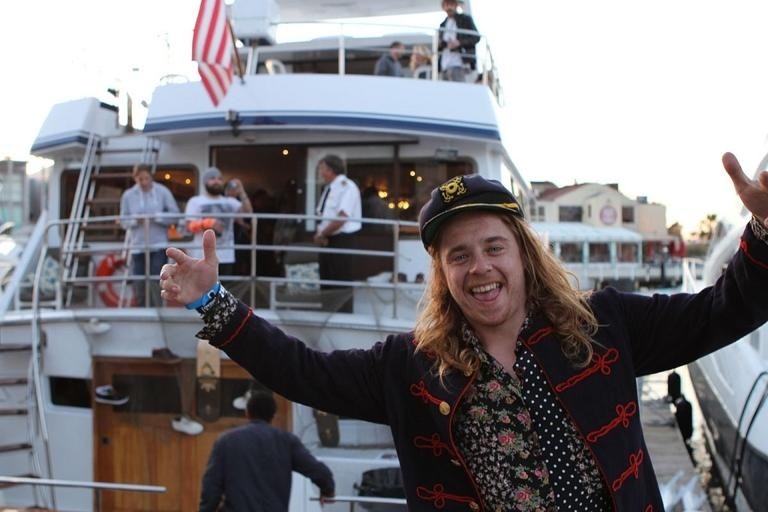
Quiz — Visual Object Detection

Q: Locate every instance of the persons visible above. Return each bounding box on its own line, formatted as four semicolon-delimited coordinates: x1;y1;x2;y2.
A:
185;167;254;287
312;152;365;311
197;389;335;512
157;149;768;512
118;163;181;309
374;0;479;83
361;186;391;231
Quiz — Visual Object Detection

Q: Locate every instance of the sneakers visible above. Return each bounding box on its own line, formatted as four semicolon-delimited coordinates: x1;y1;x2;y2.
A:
96;385;129;405
232;393;250;410
83;321;111;336
171;417;204;437
152;348;182;366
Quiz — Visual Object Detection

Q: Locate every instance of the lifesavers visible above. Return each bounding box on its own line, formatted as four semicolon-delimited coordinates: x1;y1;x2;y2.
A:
96;255;138;308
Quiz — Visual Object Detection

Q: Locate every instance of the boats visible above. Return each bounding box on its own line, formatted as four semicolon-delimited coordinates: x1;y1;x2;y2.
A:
679;152;768;510
1;0;538;512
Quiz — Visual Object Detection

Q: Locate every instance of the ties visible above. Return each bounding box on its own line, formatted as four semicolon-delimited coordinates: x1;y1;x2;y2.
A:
317;187;333;225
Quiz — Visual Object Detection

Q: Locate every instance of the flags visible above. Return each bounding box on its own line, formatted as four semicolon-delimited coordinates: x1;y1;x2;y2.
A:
192;0;235;108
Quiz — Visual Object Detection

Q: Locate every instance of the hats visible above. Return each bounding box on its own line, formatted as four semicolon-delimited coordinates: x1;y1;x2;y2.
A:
418;174;523;244
